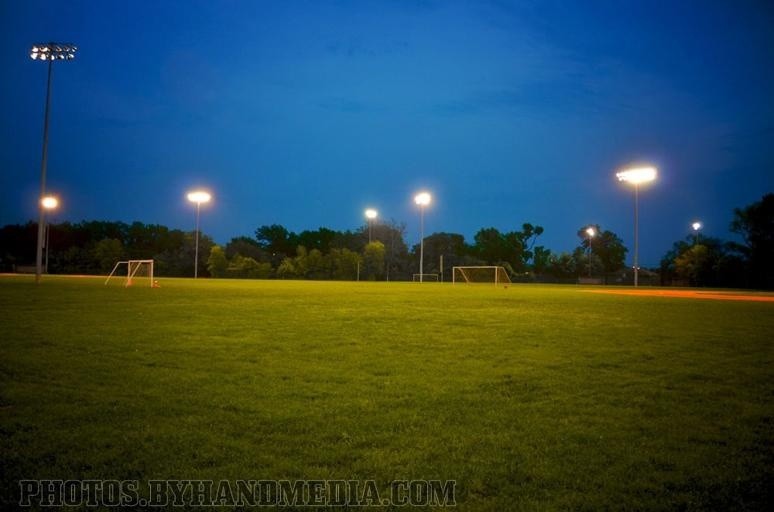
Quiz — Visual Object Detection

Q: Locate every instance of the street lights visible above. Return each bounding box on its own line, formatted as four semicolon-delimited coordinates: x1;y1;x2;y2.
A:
29;40;77;286
413;191;431;283
187;190;213;278
615;166;657;287
584;227;596;277
364;208;378;244
691;221;702;288
